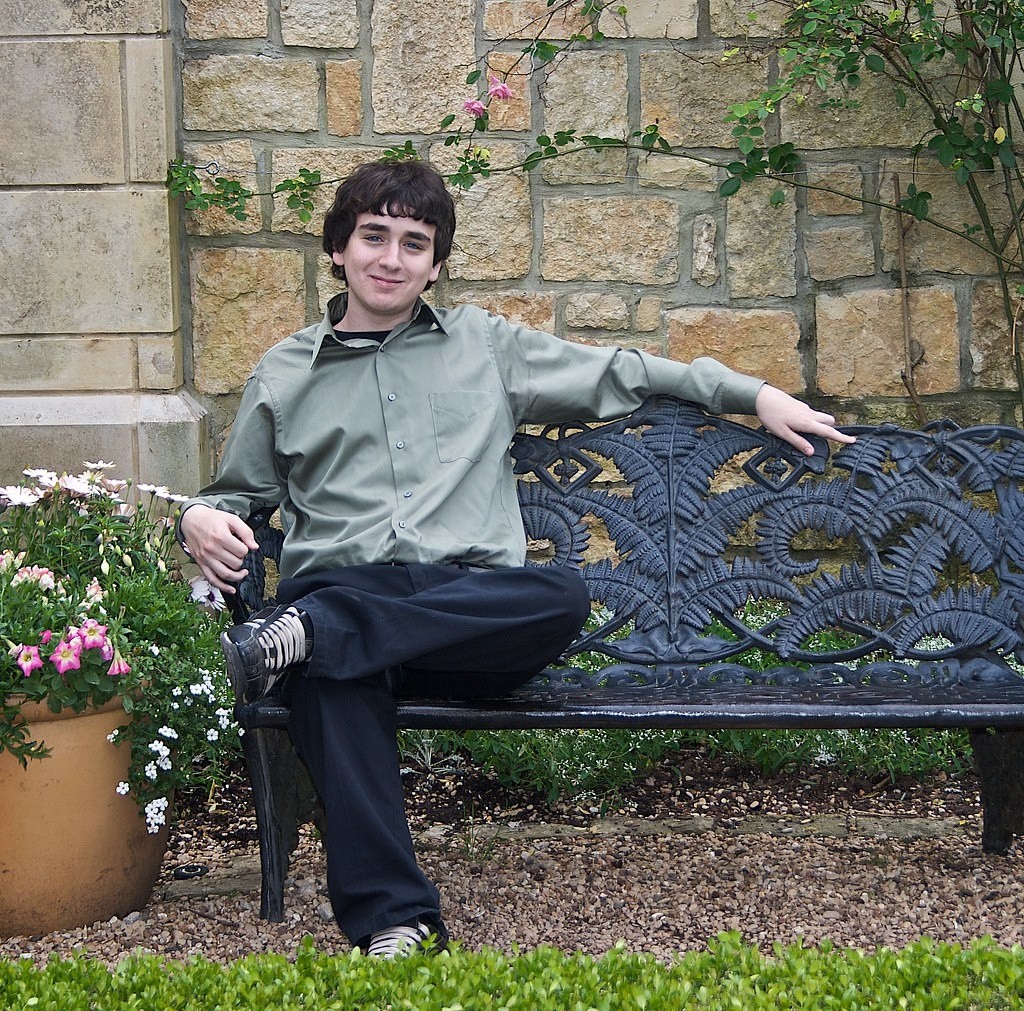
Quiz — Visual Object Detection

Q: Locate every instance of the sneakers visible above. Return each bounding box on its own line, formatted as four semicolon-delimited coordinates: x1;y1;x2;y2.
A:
363;914;447;964
219;604;315;710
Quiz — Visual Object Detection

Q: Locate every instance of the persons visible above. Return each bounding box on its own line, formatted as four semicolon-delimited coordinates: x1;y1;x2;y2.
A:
176;160;856;959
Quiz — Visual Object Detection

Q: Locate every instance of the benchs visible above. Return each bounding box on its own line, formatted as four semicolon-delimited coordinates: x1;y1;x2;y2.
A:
221;390;1024;927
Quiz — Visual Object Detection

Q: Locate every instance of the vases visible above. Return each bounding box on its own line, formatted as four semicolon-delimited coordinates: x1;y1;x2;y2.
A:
0;680;172;945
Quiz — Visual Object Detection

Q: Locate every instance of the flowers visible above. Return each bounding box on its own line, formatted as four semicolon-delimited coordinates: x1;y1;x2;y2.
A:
0;459;245;837
163;0;1022;390
